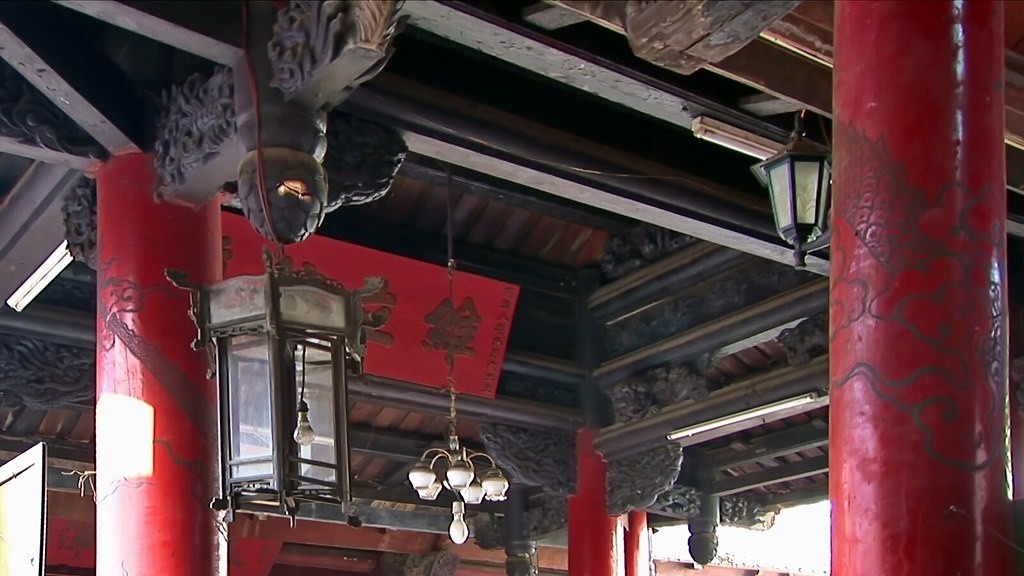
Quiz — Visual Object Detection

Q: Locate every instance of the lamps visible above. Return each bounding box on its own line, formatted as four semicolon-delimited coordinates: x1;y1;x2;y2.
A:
688;115;786;158
163;246;388;529
7;238;76;314
406;166;509;543
665;389;821;440
750;110;831;269
